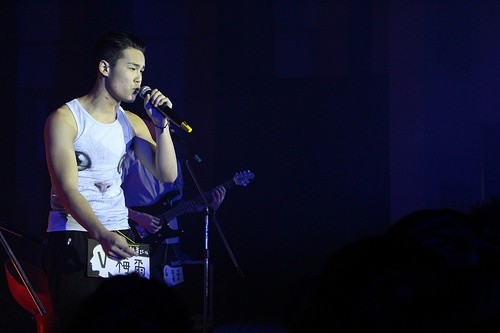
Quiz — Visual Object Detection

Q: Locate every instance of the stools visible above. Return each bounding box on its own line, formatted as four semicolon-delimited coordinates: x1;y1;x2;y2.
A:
3;256;60;333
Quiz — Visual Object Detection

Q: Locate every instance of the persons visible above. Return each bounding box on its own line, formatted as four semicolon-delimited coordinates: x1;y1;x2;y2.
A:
122;106;227;288
43;36;179;333
61;209;500;333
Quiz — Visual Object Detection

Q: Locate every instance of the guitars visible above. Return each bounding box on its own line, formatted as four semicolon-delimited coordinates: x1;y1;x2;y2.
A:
126;170;256;249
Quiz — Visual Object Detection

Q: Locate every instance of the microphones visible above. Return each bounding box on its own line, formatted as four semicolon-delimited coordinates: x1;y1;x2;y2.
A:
139;85;192;133
